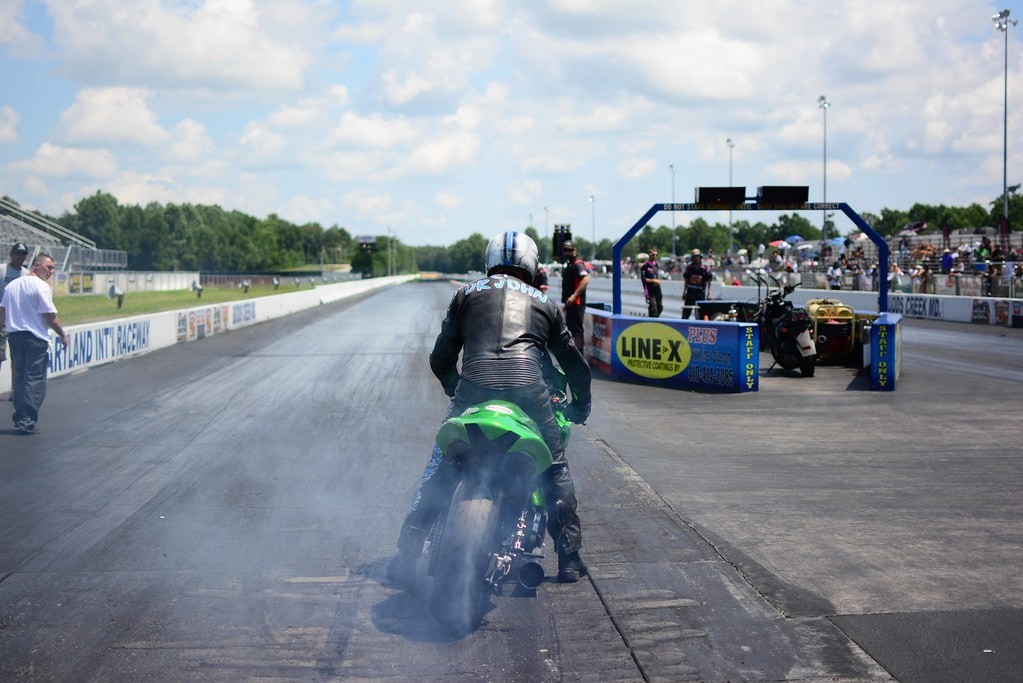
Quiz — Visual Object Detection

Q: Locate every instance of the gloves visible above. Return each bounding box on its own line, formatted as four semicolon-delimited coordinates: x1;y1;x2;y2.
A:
563;399;588;424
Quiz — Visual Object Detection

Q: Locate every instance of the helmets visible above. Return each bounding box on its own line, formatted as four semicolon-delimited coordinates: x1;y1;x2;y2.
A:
484;231;538;284
951;253;959;259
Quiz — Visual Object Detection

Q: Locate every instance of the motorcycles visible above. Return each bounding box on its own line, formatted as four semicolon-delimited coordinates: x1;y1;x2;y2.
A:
416;362;588;636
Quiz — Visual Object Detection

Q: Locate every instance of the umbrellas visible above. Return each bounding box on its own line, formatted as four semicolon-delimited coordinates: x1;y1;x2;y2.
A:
785;235;803;243
898;229;917;236
635;253;649;259
769;235;792;249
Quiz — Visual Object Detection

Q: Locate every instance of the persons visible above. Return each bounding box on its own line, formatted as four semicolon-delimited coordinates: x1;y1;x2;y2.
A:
621;235;1023;297
561;240;589;357
0;253;68;433
533;263;549;294
386;231;591;583
641;249;664;318
681;249;713;320
0;243;30;401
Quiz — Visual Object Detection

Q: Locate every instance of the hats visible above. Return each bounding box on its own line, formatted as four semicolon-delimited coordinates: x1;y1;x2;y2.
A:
562;240;575;248
12;243;29;254
692;249;701;256
944;249;950;252
647;250;657;255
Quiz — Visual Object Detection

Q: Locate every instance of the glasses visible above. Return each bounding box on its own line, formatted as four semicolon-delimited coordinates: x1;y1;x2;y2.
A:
564;248;574;253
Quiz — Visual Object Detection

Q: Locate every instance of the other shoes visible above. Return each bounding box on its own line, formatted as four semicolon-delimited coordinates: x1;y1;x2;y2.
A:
559;553;587;582
388;561;416;584
15;416;40;433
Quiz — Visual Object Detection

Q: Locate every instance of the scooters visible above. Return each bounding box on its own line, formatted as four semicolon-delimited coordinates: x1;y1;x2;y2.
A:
754;280;818;379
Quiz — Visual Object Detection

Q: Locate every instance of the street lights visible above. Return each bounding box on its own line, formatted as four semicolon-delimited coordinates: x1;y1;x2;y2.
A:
670;164;675;255
544;208;549;266
590;195;596;260
992;8;1018;218
726;137;733;258
817;93;831;240
